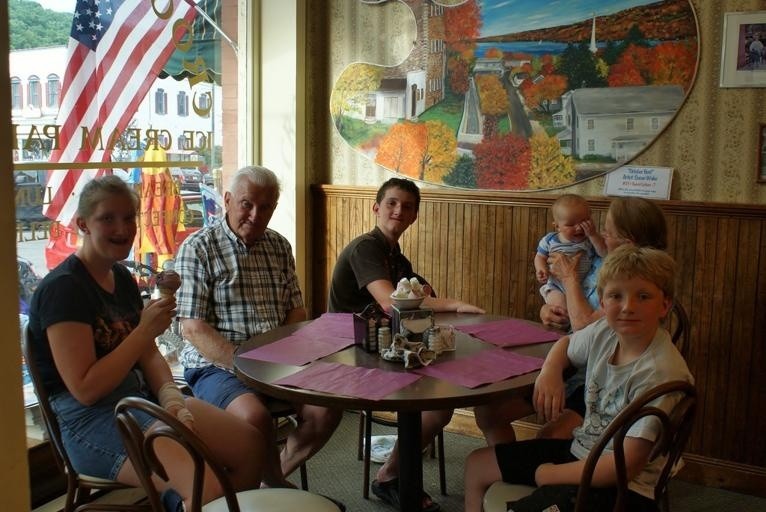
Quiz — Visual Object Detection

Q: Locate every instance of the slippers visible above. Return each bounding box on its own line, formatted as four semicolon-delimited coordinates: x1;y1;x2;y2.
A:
370;475;442;509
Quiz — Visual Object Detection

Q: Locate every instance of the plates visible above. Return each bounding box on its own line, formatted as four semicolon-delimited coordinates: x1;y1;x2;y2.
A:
363;434;397;463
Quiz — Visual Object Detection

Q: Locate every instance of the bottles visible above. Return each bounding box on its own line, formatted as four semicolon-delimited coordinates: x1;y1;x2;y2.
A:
400;277;422;296
363;317;443;355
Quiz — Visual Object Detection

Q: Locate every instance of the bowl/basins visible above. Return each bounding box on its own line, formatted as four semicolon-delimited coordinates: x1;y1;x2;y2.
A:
390;294;425;310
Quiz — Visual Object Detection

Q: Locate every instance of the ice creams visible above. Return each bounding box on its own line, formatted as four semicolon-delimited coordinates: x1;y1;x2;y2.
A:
155;269;180;301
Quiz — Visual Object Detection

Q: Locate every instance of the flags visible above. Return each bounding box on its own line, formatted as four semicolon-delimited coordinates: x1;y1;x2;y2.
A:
42;0;199;235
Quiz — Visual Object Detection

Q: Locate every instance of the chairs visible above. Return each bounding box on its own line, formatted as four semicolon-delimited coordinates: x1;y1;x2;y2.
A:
262;391;307;491
116;396;347;512
25;323;152;512
574;378;698;512
358;410;446;499
664;297;690;363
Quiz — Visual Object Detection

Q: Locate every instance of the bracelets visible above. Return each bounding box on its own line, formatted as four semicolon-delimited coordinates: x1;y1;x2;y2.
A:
157;383;185;409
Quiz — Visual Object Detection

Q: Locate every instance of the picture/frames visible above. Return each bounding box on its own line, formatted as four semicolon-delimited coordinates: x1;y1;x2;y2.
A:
720;10;766;89
756;124;766;183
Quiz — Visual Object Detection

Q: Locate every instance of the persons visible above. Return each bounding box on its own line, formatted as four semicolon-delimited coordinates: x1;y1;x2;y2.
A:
172;166;343;490
23;174;266;512
533;193;611;309
463;244;696;512
327;177;455;510
473;196;668;448
